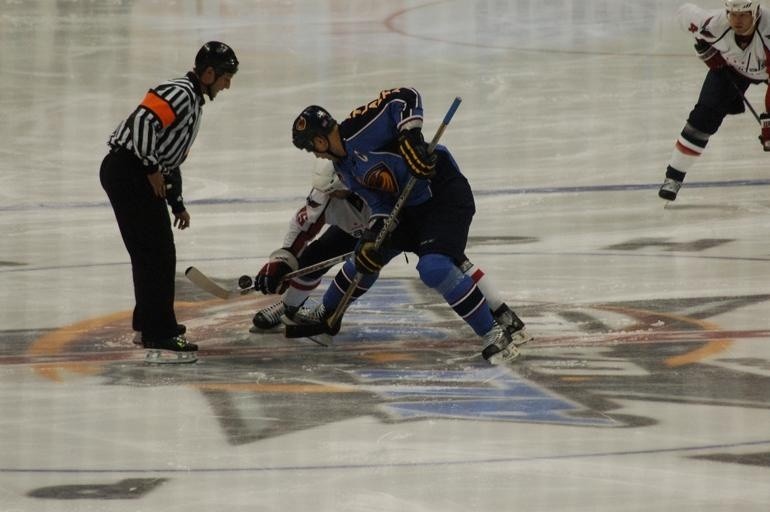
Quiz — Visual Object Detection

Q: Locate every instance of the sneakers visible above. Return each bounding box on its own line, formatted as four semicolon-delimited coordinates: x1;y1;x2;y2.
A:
143;327;199;352
493;303;524;333
658;165;686;201
480;311;513;358
132;308;188;335
252;298;310;330
288;303;335;326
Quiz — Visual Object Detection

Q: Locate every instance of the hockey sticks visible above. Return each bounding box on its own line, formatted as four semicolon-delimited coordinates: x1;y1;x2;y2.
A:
283;97;462;338
185;251;355;301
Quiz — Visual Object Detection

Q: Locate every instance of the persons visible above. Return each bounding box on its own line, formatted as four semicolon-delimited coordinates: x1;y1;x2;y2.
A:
659;1;770;201
284;84;526;360
98;40;241;354
254;156;525;334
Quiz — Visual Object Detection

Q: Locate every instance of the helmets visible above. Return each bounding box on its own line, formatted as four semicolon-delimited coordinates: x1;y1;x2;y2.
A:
290;105;336;155
724;0;762;36
311;157;350;195
193;39;239;80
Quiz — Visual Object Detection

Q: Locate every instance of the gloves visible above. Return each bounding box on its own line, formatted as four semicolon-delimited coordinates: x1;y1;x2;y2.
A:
396;128;438;181
758;114;770;153
354;232;385;275
251;249;299;297
694;41;729;71
